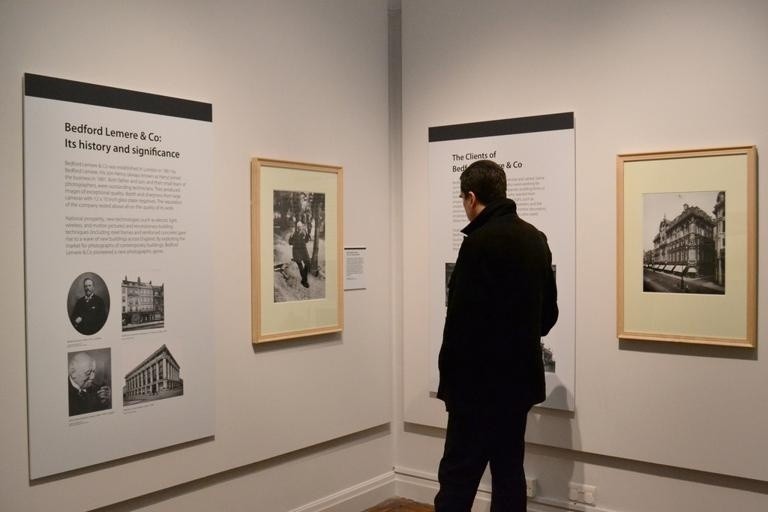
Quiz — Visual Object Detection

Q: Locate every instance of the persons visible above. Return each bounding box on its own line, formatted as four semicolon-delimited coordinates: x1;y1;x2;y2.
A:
68;352;111;416
289;221;311;288
434;159;558;512
70;277;106;335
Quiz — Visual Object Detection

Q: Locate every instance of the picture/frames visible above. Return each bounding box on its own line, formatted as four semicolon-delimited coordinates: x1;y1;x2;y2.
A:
615;145;758;349
250;156;343;346
